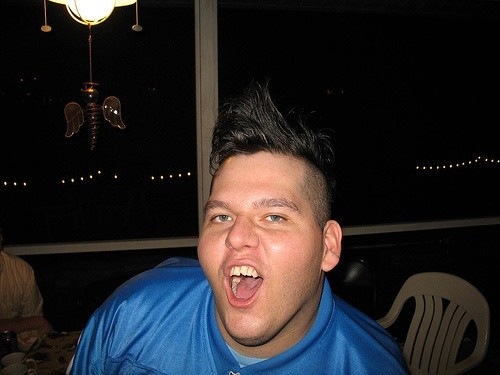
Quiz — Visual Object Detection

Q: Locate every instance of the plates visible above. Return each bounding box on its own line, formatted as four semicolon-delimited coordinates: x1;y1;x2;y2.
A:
17;326;47;351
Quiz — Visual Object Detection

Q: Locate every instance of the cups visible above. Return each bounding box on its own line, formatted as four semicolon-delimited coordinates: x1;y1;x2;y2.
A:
0;329;18;358
0;352;38;375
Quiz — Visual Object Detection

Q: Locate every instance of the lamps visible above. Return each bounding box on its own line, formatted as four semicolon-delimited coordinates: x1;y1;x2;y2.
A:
40;0;144;151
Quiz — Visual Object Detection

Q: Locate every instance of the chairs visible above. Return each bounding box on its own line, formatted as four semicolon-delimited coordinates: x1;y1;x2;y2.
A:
374;271;491;375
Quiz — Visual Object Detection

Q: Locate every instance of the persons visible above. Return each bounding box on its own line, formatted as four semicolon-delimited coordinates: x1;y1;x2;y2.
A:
0;250;54;335
66;82;411;375
